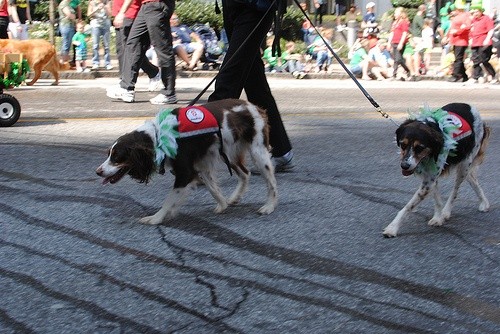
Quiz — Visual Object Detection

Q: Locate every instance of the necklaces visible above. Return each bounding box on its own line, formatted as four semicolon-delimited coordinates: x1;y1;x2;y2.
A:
392;19;400;27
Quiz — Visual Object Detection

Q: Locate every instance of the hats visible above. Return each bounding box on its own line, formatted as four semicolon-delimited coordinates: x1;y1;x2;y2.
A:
454;0;467;11
367;2;375;7
470;1;486;12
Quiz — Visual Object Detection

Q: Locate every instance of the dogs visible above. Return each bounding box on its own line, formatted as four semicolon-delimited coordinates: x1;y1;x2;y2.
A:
95;99;279;224
383;102;491;238
0;38;60;85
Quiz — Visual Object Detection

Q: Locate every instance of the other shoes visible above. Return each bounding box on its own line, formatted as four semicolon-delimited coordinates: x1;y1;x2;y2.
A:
292;71;301;79
299;71;309;79
250;149;295;174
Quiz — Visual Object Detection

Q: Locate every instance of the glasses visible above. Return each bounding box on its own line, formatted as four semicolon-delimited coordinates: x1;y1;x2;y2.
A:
170;16;179;20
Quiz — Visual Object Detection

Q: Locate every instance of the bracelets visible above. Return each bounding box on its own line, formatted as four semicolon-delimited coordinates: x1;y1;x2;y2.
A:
314;2;318;4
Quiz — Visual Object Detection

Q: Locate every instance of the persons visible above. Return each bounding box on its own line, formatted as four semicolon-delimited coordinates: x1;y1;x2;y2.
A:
262;0;414;80
209;0;296;174
0;0;33;39
105;1;179;105
58;0;205;72
409;0;500;84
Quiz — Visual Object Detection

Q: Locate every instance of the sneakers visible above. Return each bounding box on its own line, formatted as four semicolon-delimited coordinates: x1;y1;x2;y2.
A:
149;93;179;104
105;84;136;102
106;64;115;70
147;66;163;91
91;63;100;70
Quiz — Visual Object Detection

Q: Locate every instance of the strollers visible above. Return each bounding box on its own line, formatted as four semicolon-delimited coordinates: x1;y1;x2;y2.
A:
189;23;223;70
